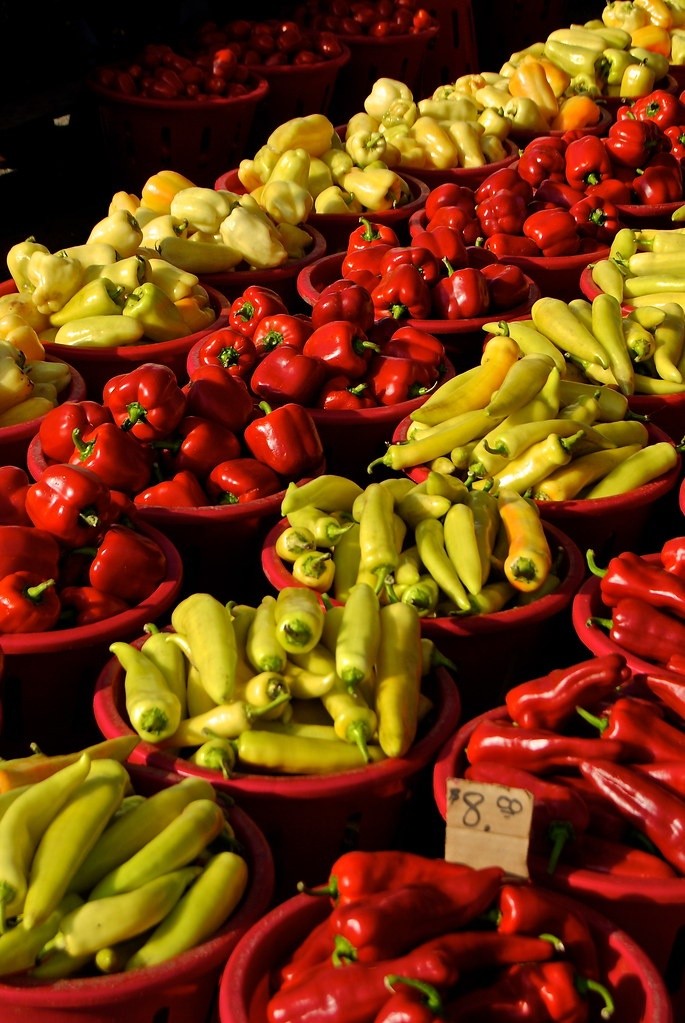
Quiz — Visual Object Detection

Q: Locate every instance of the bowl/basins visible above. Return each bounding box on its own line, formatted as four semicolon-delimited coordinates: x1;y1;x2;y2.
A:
1;0;685;1023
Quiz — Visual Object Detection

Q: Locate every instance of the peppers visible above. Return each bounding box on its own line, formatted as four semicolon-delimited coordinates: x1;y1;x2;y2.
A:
0;0;685;1023
